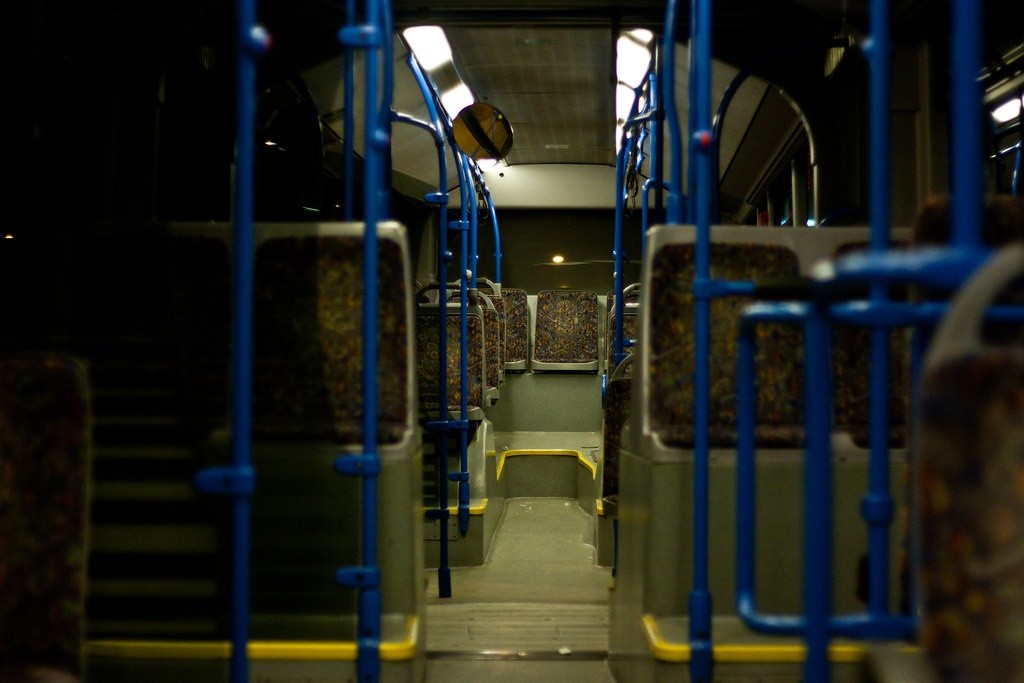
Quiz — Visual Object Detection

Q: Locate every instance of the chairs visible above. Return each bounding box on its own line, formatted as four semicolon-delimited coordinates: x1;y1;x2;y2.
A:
0;216;1024;683
